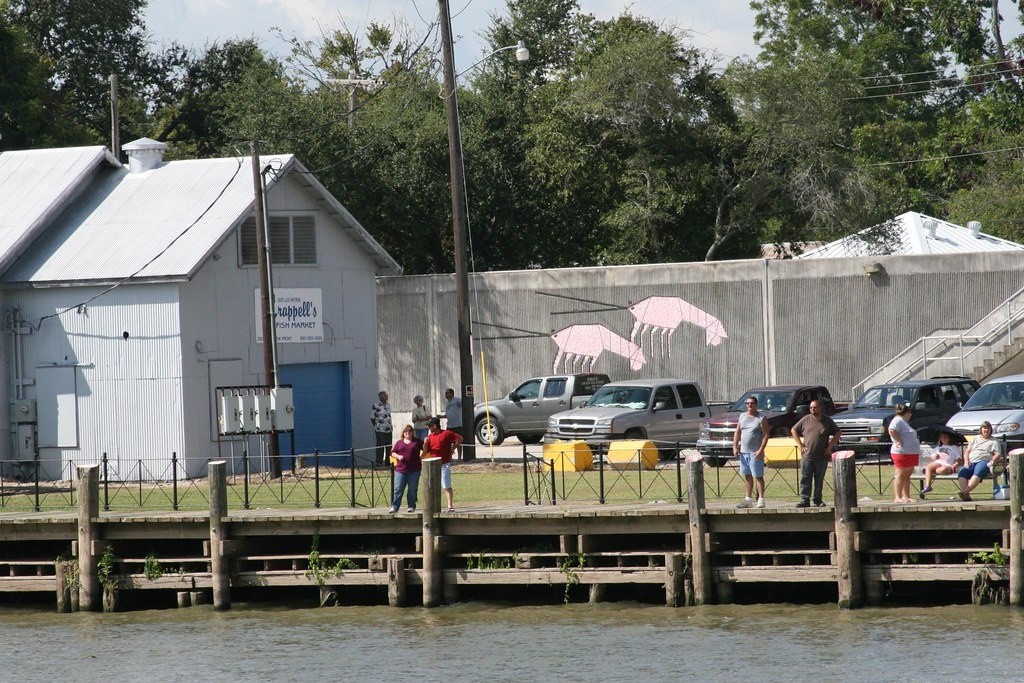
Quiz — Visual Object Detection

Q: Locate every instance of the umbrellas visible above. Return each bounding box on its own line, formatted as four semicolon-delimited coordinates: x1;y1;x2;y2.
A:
916;423;968;454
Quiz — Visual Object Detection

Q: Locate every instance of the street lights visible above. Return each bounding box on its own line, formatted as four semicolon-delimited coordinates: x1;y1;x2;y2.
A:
442;41;529;463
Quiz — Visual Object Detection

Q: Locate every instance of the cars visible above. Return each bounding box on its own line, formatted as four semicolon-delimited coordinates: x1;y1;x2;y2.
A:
946;374;1024;456
828;374;981;461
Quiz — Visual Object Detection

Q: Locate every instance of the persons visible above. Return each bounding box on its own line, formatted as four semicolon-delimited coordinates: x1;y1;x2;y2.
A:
423;417;463;511
437;388;462;461
388;424;424;513
370;391;393;466
921;431;963;494
957;421;1001;501
733;396;769;508
412;395;432;441
888;404;920;503
791;400;842;508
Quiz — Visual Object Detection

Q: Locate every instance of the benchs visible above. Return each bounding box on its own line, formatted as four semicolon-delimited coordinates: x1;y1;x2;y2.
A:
914;433;1008;500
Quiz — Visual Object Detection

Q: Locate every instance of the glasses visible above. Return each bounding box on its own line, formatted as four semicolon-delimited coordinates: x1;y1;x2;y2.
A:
404;430;412;432
746;401;755;405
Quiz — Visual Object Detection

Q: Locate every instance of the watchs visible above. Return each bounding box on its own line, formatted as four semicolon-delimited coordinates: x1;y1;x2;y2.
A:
830;443;836;447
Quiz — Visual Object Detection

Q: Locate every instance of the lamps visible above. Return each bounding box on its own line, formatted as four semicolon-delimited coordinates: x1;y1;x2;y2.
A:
864;262;883;274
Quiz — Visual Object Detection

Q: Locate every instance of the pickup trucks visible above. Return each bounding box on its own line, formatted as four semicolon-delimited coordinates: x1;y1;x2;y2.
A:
697;385;849;468
474;373;613;447
543;378;736;461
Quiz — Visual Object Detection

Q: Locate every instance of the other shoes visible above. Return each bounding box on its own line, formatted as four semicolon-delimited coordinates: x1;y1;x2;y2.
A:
812;500;826;507
757;498;765;508
736;498;754;509
958;491;973;502
895;497;918;504
795;499;810;508
407;507;415;512
389;508;399;513
921;487;933;493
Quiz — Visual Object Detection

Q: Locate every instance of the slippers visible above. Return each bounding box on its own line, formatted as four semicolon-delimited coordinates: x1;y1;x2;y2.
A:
448;508;454;512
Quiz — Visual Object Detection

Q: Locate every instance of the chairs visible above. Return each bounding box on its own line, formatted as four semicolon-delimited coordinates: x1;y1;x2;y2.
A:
892;395;903;406
944;390;956;400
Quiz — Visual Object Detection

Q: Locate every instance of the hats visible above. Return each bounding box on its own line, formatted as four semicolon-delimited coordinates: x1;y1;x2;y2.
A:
424;417;441;425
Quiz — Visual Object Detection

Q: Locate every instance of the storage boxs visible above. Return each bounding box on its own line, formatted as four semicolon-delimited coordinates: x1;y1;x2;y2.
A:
992;484;1010;499
